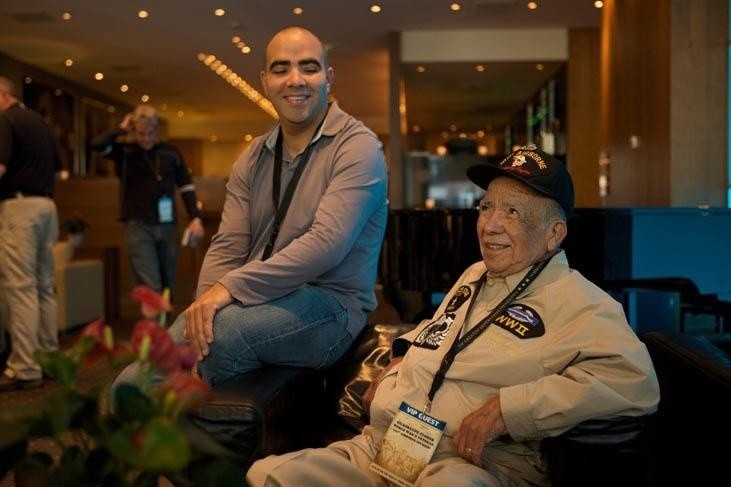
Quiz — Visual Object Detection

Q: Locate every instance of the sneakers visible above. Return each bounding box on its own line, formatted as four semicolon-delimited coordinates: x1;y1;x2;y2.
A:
0;372;44;392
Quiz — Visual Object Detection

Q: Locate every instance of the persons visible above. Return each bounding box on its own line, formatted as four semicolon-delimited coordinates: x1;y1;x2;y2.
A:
0;76;60;391
98;104;204;312
156;28;389;383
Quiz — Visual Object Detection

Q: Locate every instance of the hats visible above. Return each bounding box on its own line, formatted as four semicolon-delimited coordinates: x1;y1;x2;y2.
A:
465;144;574;218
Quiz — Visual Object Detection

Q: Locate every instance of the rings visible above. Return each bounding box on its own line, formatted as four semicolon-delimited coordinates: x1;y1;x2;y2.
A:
466;447;472;452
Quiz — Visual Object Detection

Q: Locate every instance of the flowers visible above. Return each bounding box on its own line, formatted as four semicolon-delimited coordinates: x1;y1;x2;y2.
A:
0;287;242;486
